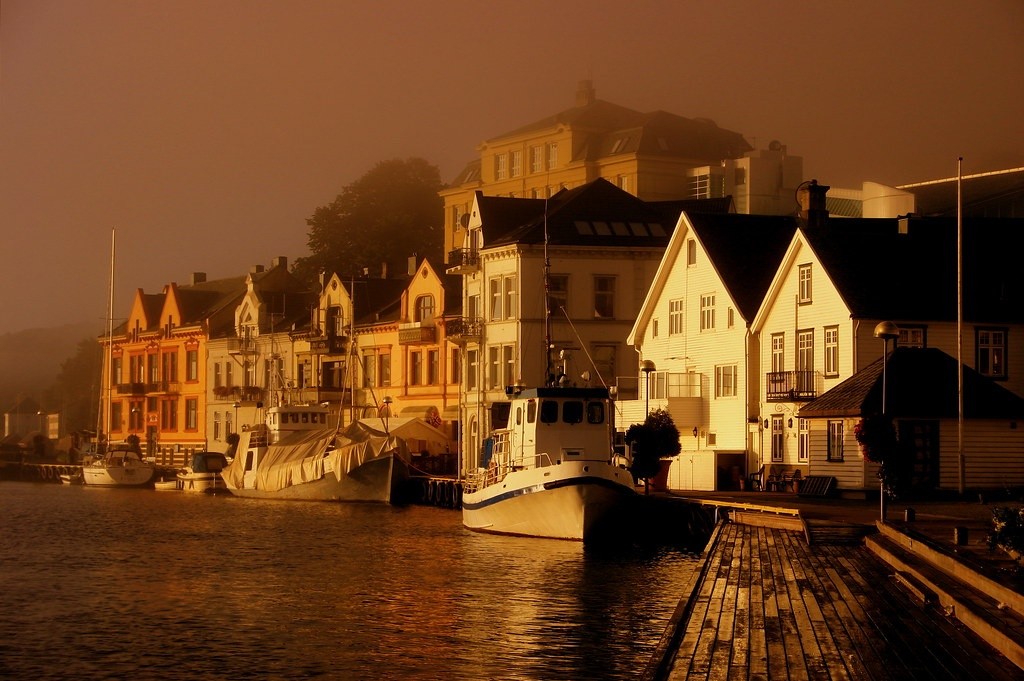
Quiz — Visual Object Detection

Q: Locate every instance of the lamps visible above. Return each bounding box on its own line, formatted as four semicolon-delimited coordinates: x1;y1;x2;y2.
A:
764;419;768;429
693;427;697;438
788;418;792;428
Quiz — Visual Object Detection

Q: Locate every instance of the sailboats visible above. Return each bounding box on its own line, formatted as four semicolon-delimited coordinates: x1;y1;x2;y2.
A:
82;226;153;487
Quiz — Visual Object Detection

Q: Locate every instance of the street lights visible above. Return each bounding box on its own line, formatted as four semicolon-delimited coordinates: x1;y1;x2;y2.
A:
233;401;241;434
38;410;45;432
640;360;656;494
132;407;140;435
874;320;900;523
382;396;393;431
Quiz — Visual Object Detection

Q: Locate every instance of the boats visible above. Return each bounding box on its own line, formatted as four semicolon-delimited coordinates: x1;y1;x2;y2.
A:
155;478;177;490
60;474;81;485
220;274;411;504
462;189;638;542
175;452;228;494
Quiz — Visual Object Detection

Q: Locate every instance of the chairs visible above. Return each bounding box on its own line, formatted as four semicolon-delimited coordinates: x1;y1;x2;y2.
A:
749;466;765;491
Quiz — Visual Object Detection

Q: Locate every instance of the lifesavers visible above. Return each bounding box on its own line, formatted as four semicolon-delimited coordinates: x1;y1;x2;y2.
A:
421;480;463;507
35;465;78;480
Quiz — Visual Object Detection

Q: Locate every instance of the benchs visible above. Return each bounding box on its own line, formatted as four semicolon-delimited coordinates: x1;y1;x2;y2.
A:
767;468;801;492
